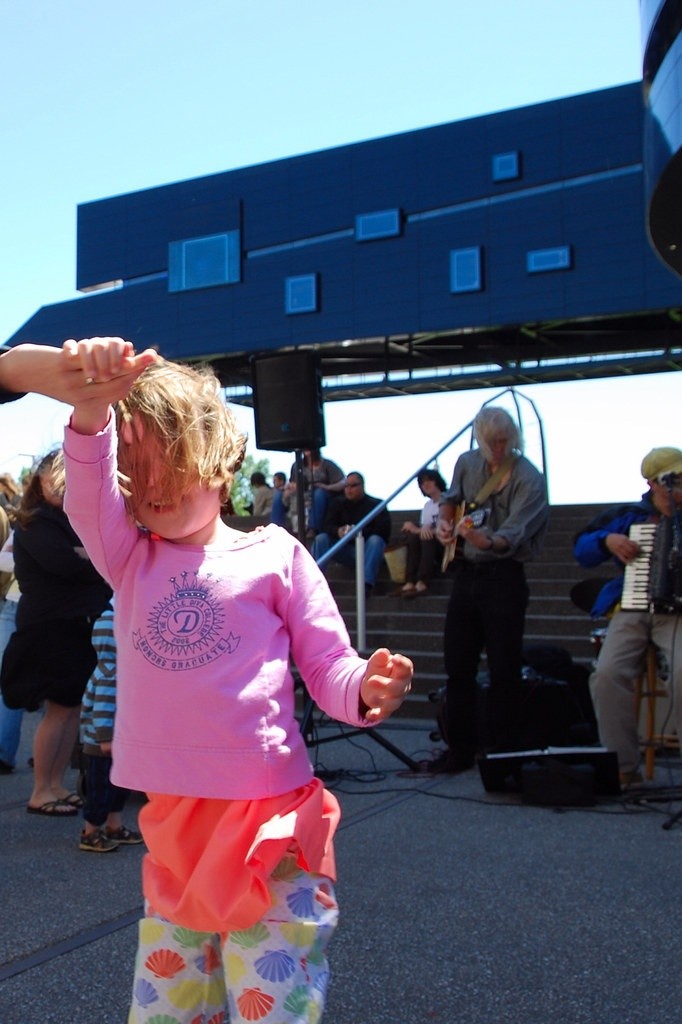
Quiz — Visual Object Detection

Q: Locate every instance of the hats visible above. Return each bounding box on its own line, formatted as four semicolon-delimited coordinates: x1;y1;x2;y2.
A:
641;447;682;482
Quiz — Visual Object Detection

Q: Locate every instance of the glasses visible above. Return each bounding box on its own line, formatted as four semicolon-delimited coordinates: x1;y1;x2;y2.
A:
344;482;360;488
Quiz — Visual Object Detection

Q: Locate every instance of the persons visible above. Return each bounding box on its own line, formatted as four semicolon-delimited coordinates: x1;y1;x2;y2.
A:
389;468;450;595
270;473;289;529
572;447;682;789
280;446;346;537
434;403;547;769
0;447;116;816
220;496;238;517
0;343;158;407
309;472;391;597
60;336;417;1024
248;472;274;515
73;599;145;853
280;473;312;532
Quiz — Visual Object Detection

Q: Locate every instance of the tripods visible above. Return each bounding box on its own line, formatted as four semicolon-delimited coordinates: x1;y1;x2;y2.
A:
634;485;682;829
292;450;419;774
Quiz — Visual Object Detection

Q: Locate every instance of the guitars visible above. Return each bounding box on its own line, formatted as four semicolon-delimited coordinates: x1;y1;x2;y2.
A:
441;498;489;581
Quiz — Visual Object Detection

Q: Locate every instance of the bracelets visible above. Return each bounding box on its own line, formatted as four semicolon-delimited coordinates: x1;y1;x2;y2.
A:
481;537;494;550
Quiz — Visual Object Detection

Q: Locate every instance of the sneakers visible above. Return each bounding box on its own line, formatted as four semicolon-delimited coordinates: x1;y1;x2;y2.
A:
105;825;143;843
79;829;118;852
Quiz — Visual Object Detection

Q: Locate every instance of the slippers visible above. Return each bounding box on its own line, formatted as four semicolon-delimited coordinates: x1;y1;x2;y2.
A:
27;799;78;816
60;793;84;808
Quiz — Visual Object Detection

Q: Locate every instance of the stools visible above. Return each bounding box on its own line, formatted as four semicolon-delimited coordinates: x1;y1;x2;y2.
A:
606;601;681;781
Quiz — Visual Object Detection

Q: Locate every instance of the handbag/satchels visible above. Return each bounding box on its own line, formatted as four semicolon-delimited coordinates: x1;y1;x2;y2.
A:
384;538;408;584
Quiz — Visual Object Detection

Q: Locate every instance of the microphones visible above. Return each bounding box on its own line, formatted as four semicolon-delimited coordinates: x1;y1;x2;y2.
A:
658;470;678;484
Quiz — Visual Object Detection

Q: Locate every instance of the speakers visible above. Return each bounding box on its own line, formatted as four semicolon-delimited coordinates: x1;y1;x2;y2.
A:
250;348;326;452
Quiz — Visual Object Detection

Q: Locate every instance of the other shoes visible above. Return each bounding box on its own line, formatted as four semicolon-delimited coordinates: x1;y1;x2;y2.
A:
618;769;644;790
403;583;432;598
306;529;317;539
420;745;474;774
0;762;12;774
365;584;371;597
386;585;415;597
